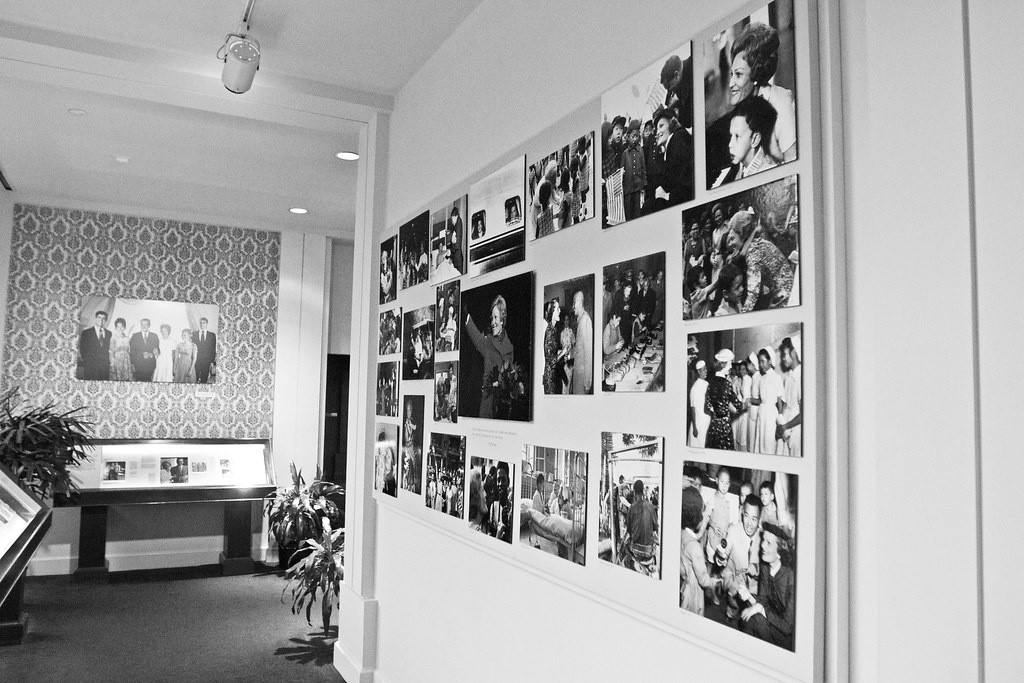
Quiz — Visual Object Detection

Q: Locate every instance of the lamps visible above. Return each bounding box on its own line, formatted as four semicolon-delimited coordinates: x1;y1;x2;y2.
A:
218;32;265;94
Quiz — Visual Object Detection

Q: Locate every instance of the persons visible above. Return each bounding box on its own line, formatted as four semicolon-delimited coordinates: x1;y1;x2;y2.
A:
195;317;216;384
80;311;112;381
153;323;177;383
470;130;594;238
468;448;588;564
459;294;525;422
129;318;160;381
543;288;591;396
108;316;133;381
171;457;188;483
103;463;122;479
372;196;464;529
600;21;792;650
173;329;198;383
161;462;174;483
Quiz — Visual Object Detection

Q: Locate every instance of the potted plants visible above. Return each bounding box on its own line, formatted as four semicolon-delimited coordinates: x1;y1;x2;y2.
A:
254;460;345;568
0;386;100;646
